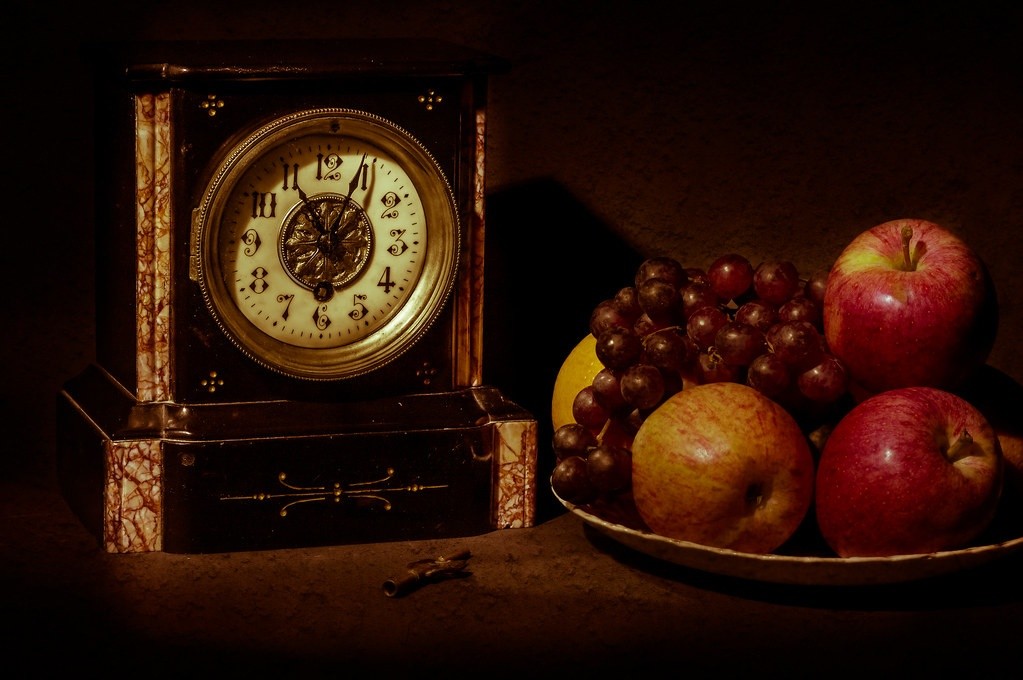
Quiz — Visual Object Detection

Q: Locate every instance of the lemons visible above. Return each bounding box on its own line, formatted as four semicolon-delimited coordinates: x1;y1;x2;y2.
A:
552;333;605;430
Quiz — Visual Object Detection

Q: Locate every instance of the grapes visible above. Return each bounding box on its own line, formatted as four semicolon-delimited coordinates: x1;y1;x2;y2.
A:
552;252;847;507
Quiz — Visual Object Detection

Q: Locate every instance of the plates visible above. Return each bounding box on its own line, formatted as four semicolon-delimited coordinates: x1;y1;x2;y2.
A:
549;479;1023;587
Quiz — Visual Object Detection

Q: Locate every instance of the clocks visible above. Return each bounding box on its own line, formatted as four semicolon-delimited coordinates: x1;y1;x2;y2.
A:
56;42;539;553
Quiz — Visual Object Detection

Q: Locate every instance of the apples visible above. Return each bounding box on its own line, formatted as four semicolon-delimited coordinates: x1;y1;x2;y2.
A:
824;219;985;391
815;387;1023;557
632;382;814;554
